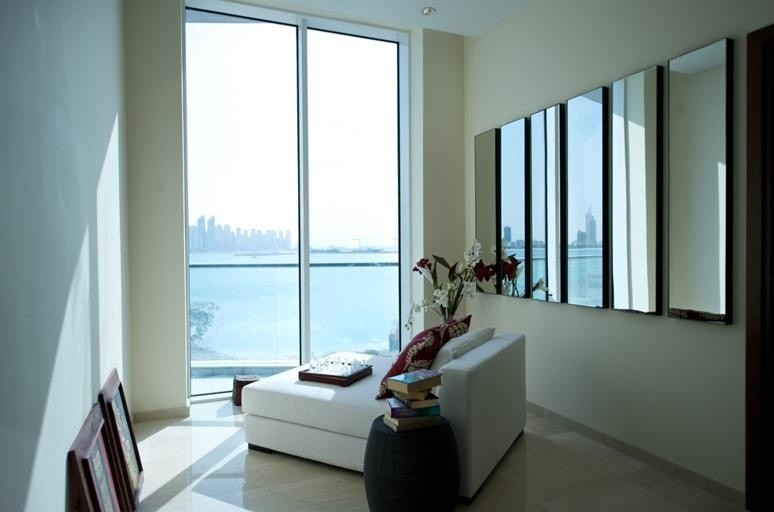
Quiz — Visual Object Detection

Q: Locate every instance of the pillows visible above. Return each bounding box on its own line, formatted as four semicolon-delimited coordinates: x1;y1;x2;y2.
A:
376;314;496;403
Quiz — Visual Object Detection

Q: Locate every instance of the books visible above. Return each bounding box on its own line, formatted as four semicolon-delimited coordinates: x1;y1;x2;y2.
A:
382;369;444;433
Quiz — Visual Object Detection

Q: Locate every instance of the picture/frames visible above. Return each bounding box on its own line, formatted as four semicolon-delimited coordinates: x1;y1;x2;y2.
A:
66;367;143;512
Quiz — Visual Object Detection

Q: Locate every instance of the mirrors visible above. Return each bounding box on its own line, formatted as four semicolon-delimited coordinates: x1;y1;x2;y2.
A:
471;102;565;304
601;66;663;314
563;87;609;309
664;36;734;324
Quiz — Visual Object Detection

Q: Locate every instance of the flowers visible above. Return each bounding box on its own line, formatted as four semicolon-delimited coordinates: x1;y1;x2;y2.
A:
403;236;486;330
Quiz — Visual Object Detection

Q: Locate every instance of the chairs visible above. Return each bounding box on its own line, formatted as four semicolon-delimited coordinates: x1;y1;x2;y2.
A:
240;330;527;507
